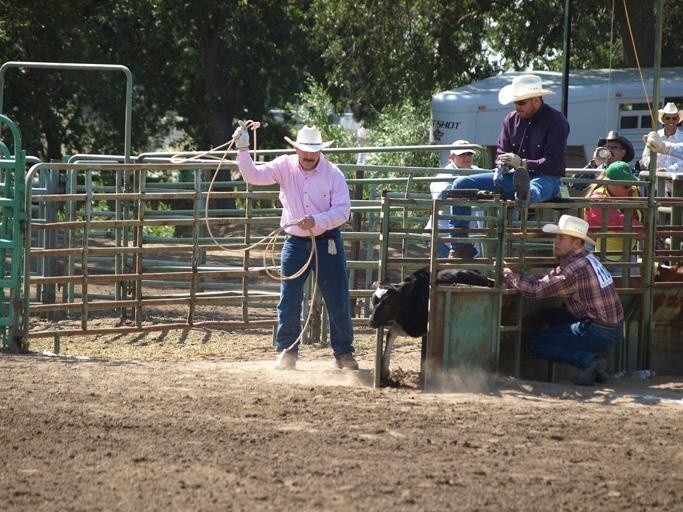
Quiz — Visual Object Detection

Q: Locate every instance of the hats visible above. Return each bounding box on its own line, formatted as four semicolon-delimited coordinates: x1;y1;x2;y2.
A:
606;160;640;181
597;131;635;162
283;124;334;152
658;102;683;124
450;140;476;155
498;74;556;105
541;214;597;245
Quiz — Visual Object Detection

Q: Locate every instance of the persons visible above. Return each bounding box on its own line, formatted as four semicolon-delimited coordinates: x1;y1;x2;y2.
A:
493;215;625;385
646;131;683;160
584;161;670;277
446;75;569;264
638;103;683;248
234;125;359;372
570;130;650;197
422;140;489;266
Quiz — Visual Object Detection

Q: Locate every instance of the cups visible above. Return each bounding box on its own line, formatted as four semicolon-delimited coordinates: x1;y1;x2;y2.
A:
597;149;611;158
641;132;658;145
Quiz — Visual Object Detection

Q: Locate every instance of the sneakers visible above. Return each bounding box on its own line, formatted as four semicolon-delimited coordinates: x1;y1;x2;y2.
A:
275;352;296;370
335;354;358;370
448;243;478;258
513;167;530;211
573;356;608;384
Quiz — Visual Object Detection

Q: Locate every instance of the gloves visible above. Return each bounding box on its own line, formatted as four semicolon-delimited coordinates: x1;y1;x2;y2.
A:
493;153;527;195
646;130;669;154
232;120;250;148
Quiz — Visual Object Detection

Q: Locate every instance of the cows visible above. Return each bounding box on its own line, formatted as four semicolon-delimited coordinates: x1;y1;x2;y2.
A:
368;264;494;383
